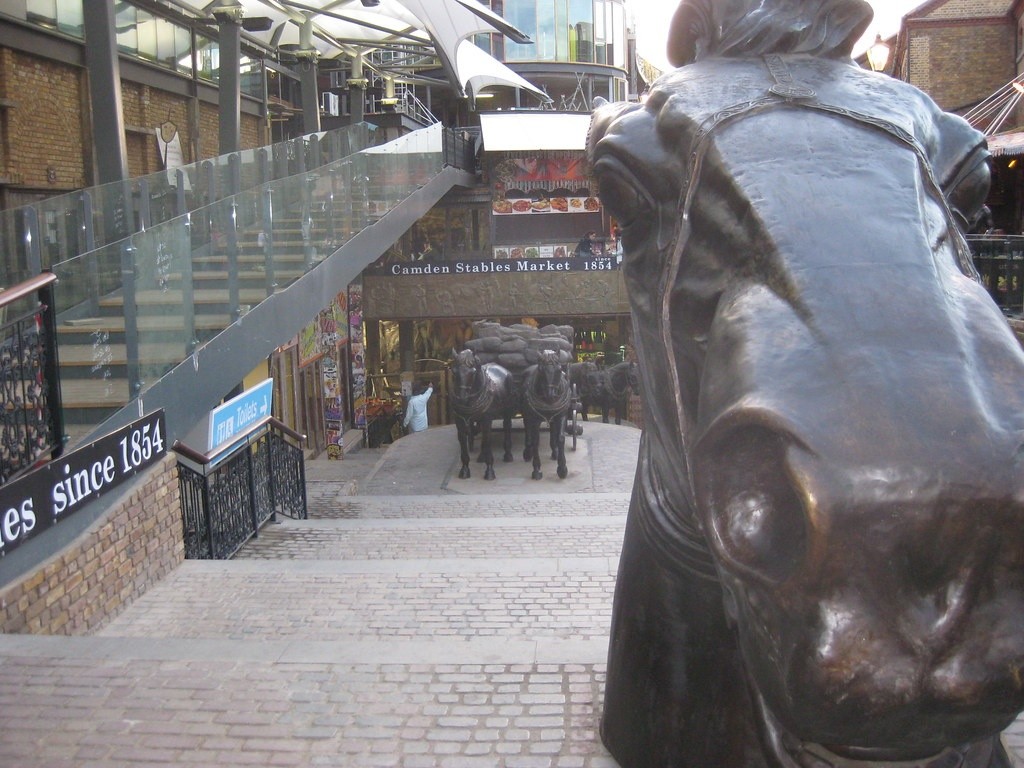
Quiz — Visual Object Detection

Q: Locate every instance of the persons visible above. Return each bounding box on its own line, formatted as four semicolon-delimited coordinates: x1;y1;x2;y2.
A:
609;229;623;265
968;204;994;260
403;382;433;434
574;229;598;258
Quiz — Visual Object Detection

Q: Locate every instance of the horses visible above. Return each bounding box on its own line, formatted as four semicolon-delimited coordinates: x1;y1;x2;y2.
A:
453;348;570;479
600;3;1021;755
570;360;641;425
